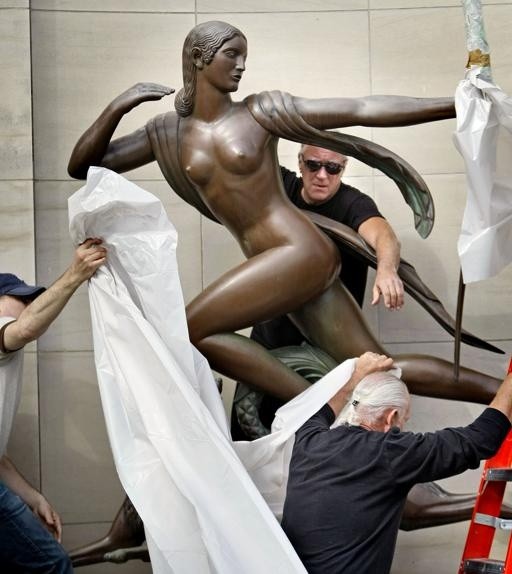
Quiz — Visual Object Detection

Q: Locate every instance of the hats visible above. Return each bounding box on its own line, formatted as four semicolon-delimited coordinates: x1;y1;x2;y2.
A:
0;273;46;303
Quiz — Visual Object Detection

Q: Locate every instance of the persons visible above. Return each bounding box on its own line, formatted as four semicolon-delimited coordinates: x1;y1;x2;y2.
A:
230;144;405;440
281;351;512;574
68;21;504;401
0;239;106;574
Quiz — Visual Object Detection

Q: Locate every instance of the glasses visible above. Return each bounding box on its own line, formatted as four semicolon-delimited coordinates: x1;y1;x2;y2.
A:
300;154;345;175
